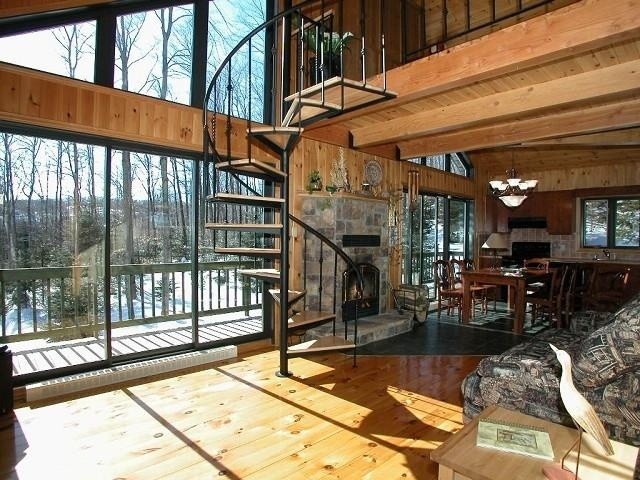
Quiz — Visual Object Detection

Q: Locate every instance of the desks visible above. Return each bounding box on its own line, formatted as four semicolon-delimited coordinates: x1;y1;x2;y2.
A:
430;404;640;480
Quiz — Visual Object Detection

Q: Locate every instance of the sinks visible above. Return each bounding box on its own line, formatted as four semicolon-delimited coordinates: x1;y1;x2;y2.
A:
597;258;619;262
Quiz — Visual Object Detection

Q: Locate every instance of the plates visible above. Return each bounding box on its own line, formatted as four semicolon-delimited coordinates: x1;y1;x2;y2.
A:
365;159;383;186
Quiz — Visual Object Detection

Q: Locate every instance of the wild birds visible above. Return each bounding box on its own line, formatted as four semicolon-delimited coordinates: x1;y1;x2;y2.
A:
548;341;616;480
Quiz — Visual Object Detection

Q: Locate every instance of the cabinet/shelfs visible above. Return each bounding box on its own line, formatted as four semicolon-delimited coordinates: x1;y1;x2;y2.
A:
584;261;640;311
479;256;508;301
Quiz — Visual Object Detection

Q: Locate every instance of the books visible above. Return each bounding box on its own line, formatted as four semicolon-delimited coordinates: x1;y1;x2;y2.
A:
476;417;556;461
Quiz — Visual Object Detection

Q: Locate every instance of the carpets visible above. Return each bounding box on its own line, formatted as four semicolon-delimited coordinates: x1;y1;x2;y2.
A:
339;298;572;356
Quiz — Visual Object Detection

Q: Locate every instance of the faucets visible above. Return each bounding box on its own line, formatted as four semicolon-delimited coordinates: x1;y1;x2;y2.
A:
603;249;610;259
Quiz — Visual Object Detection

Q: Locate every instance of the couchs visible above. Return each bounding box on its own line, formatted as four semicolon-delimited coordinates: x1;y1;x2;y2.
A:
461;289;640;447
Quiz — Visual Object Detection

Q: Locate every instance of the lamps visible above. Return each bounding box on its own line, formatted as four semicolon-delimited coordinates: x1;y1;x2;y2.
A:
488;150;539;209
482;233;510;272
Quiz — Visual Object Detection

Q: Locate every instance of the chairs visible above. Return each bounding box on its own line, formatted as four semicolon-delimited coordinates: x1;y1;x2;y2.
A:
434;259;598;337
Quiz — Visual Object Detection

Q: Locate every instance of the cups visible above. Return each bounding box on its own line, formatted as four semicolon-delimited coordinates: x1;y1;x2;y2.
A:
543;260;550;274
610;253;617;261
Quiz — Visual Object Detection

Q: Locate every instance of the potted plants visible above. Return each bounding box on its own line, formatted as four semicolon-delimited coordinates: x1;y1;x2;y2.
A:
307;169;323;192
290;16;355;86
